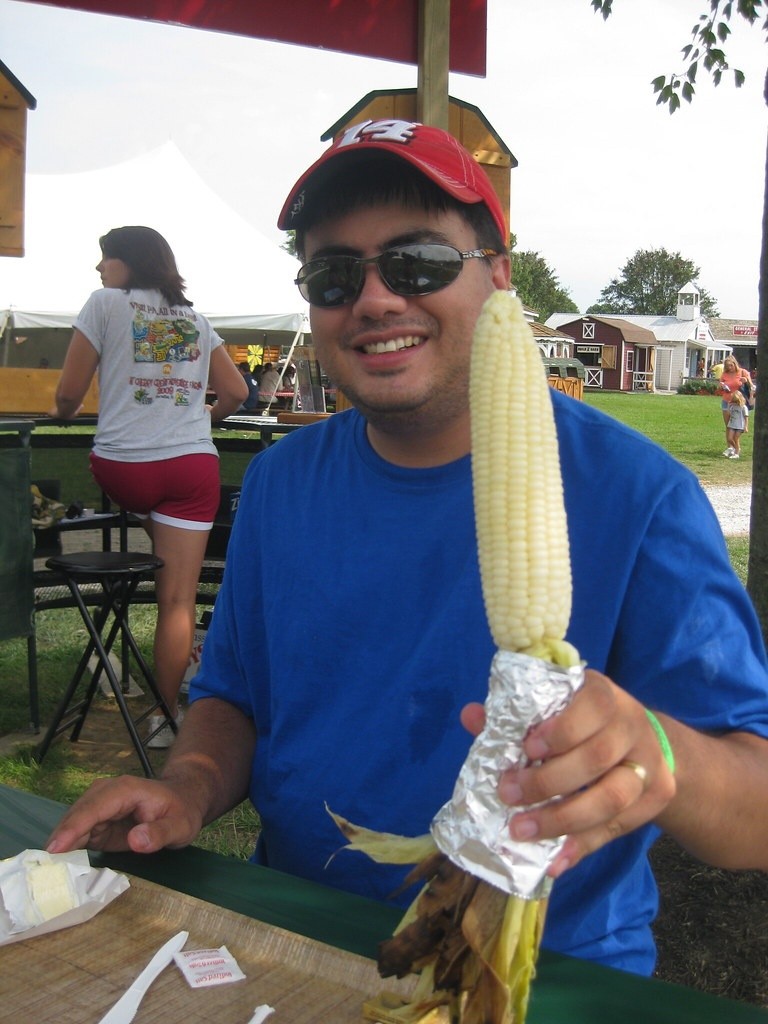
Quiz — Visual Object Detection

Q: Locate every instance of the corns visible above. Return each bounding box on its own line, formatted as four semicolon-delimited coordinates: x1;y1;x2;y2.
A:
468;288;572;654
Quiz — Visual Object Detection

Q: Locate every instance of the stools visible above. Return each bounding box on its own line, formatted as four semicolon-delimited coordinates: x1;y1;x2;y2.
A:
33;552;179;777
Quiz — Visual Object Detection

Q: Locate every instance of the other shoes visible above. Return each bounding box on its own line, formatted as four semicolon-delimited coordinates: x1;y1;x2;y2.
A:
729;454;739;459
722;447;735;456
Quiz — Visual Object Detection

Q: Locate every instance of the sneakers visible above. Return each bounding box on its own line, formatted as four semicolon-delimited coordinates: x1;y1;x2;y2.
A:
146;707;185;747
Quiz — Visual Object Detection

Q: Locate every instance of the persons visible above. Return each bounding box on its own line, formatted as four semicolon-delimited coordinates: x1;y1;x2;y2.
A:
727;389;749;459
48;225;250;747
707;356;715;378
711;360;724;379
238;362;296;410
696;358;705;377
717;355;753;457
45;117;768;978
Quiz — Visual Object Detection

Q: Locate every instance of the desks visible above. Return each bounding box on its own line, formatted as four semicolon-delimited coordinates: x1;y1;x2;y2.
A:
0;783;768;1024
202;390;297;414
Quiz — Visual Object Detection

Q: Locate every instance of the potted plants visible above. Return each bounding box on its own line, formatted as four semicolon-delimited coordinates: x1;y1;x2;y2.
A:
675;378;721;396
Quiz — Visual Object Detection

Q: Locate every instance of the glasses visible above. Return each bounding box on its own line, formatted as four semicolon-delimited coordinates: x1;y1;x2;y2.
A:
295;241;499;308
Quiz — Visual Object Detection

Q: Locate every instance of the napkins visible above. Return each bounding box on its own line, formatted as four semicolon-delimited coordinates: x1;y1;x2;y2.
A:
0;848;131;948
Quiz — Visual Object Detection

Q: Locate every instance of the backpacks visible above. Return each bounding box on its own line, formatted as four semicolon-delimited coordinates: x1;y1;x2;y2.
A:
740;380;754;410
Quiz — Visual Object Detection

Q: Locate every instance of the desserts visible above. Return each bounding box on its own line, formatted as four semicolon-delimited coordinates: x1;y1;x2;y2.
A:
24;858;77;925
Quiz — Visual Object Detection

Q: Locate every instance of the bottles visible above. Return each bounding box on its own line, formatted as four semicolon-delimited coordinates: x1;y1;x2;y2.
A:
722;383;731;392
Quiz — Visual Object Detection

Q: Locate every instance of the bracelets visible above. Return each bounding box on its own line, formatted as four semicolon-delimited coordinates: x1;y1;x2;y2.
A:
644;710;676;774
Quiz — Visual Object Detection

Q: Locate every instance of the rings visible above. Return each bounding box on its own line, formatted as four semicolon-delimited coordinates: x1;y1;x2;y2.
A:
616;760;649;790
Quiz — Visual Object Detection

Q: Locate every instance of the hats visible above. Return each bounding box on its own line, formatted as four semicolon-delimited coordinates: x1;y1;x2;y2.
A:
278;118;507;251
